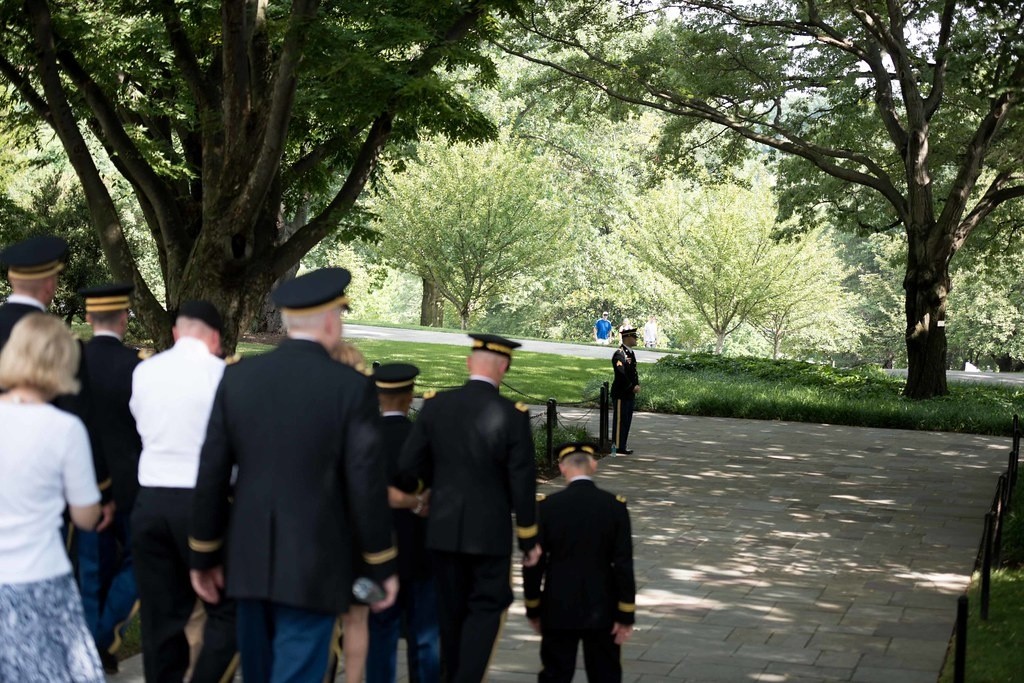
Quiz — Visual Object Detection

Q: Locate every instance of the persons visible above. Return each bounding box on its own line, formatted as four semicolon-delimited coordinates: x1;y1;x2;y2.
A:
130;265;398;683
612;328;640;454
0;236;143;683
365;334;636;683
594;311;611;346
643;315;658;348
620;318;632;345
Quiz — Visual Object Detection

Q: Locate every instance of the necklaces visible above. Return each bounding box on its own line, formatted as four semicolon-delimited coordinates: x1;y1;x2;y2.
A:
5;395;42;405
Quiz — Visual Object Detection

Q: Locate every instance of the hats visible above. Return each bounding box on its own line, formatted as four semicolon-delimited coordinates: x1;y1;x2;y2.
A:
375;364;419;393
0;236;71;280
553;442;601;463
77;283;135;312
619;329;638;339
269;268;350;316
468;334;522;371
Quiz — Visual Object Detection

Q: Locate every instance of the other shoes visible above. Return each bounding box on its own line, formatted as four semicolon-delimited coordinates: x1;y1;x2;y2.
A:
616;449;633;454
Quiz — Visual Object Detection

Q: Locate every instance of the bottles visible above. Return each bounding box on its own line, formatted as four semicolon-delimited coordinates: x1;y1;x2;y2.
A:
351;579;386;605
